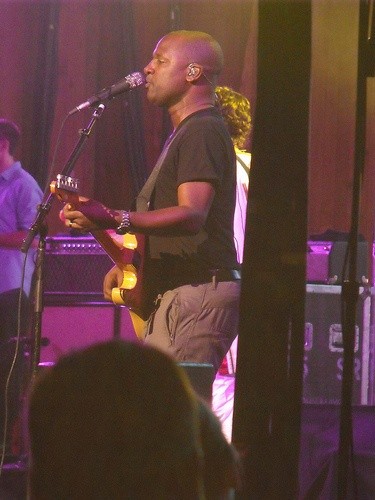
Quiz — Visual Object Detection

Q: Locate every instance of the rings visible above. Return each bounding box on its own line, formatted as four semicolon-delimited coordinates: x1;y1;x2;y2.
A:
69;221;73;229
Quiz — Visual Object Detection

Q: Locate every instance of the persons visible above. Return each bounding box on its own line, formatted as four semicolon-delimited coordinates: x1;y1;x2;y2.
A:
24;338;210;500
58;30;244;404
195;395;241;500
211;85;253;443
0;119;46;470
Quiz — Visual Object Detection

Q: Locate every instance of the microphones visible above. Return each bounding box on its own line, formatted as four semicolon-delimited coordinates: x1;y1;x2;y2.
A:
69;71;146;112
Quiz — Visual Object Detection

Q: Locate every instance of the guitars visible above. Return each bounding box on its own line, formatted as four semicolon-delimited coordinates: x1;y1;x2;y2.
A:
48;172;148;341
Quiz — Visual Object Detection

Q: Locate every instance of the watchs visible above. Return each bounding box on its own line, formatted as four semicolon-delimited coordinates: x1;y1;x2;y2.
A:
114;210;131;235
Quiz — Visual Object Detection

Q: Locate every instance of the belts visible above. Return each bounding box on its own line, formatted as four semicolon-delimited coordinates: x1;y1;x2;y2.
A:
147;269;242;291
